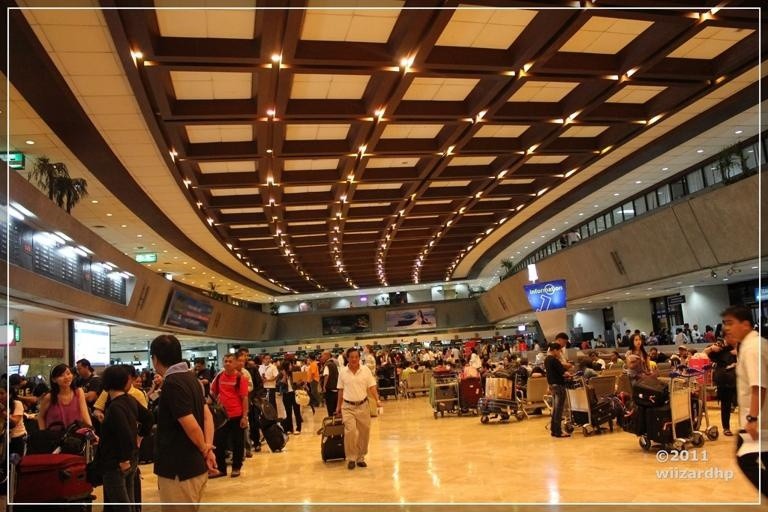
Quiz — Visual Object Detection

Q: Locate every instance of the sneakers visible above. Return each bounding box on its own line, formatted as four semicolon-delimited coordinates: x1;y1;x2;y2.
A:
208;471;228;478
245;445;262;458
550;432;571;438
287;430;301;435
230;468;240;477
348;460;367;470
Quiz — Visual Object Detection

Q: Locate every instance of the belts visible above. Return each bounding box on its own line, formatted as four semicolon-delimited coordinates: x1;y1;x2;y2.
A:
344;397;367;406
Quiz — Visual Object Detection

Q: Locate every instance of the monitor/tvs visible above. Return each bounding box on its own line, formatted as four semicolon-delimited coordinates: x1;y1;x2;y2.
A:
8;364;20;376
19;364;29;376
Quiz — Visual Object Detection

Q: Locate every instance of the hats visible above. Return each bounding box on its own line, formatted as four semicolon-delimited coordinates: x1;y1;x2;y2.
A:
678;344;687;350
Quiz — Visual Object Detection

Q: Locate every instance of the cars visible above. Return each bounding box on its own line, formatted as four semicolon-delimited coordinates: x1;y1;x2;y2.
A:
398;311;417;324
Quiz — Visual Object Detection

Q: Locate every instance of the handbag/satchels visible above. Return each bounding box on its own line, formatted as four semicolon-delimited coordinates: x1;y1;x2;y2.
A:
294;389;311;406
25;421;87;455
485;375;513;401
317;415;343;435
274;391;289;421
206;390;228;433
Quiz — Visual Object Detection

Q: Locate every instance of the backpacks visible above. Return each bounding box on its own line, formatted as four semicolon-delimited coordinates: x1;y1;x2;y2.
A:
252;397;278;429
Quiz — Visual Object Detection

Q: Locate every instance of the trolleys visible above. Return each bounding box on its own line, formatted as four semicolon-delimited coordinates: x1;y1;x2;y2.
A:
13;425;95;512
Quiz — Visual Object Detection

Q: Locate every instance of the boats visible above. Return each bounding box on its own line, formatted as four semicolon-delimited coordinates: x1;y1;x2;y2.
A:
353;320;368;330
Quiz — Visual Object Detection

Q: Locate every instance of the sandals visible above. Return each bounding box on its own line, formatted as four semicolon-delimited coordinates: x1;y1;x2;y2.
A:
723;431;733;436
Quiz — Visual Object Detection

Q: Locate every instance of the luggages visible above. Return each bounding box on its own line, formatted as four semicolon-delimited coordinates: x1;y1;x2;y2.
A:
632;376;670;407
435;383;456;412
459;377;483;410
317;411;347;463
375;363;399;395
644;406;674;443
526;376;550;404
11;453;93;502
260;423;290;453
571;399;617;426
